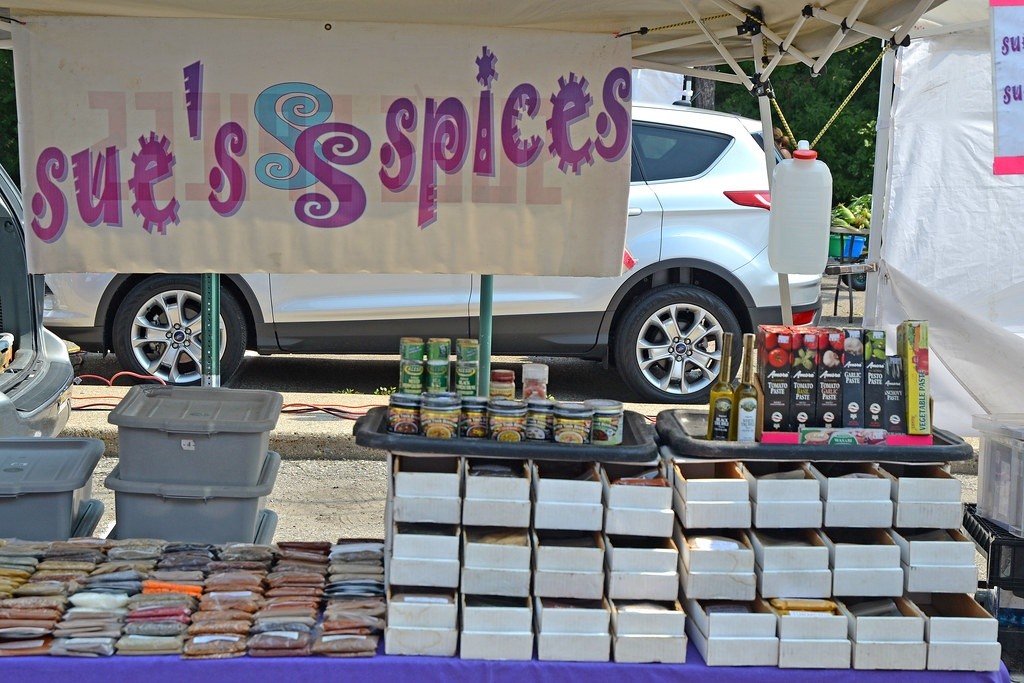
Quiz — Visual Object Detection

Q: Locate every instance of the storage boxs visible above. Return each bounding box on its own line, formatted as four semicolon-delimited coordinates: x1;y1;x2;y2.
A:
458;594;535;661
383;587;460;658
390;521;461;589
393;456;462;525
462;458;532;528
533;595;611;662
530;459;605;532
532;528;605;600
608;596;689;664
102;450;283;542
107;508;279;545
657;319;1024;683
351;406;658;462
601;463;675;539
0;436;105;538
603;534;679;601
107;384;284;486
460;527;532;597
73;498;105;537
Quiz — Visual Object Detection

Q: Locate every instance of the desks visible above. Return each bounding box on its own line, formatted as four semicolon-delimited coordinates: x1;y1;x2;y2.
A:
0;653;1012;683
831;226;870;323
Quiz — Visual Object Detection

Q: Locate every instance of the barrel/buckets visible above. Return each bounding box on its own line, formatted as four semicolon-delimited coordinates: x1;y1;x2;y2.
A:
844;236;866;257
830;235;848;256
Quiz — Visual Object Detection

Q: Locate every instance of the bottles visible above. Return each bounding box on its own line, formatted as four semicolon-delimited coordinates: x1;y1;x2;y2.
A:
767;140;833;274
708;333;735;440
729;333;758;442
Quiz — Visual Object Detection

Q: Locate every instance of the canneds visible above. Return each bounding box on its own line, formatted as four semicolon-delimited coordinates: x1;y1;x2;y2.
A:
384;337;623;446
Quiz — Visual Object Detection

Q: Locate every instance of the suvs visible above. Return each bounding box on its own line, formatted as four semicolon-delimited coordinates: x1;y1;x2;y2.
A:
0;20;826;405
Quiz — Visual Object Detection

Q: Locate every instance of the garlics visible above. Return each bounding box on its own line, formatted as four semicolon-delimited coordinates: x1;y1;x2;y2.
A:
823;351;839;367
844;337;863;355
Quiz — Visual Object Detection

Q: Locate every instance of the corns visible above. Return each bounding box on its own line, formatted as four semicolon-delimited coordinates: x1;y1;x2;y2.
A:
833;204;871;228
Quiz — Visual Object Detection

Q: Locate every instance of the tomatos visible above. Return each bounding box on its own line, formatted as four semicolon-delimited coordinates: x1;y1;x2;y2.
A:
765;332;792;367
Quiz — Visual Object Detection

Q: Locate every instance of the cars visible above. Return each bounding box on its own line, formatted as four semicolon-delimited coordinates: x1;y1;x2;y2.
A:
0;166;76;442
